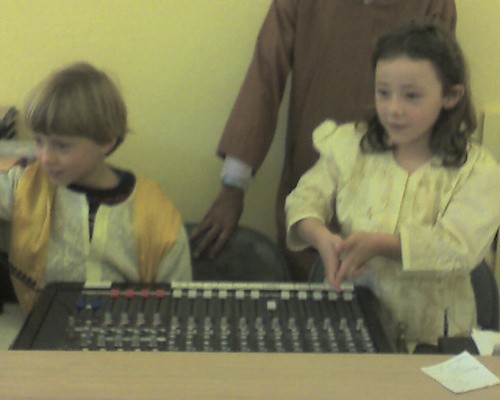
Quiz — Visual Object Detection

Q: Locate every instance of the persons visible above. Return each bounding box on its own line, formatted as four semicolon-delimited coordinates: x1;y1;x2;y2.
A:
189;0;458;285
286;24;500;342
1;62;192;312
